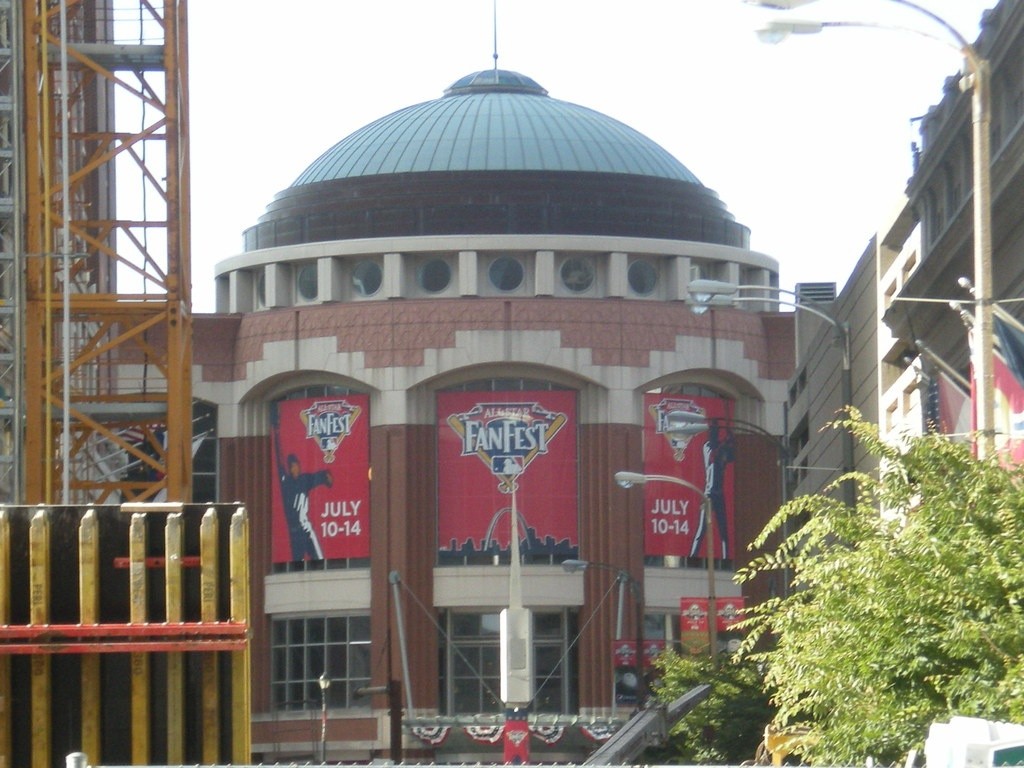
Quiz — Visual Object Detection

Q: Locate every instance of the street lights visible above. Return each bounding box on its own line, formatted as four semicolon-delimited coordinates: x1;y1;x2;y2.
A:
616;471;720;672
747;0;997;467
687;279;858;551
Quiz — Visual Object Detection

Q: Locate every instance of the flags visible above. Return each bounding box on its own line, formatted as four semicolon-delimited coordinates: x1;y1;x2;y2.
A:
913;292;1024;479
117;411;207;502
